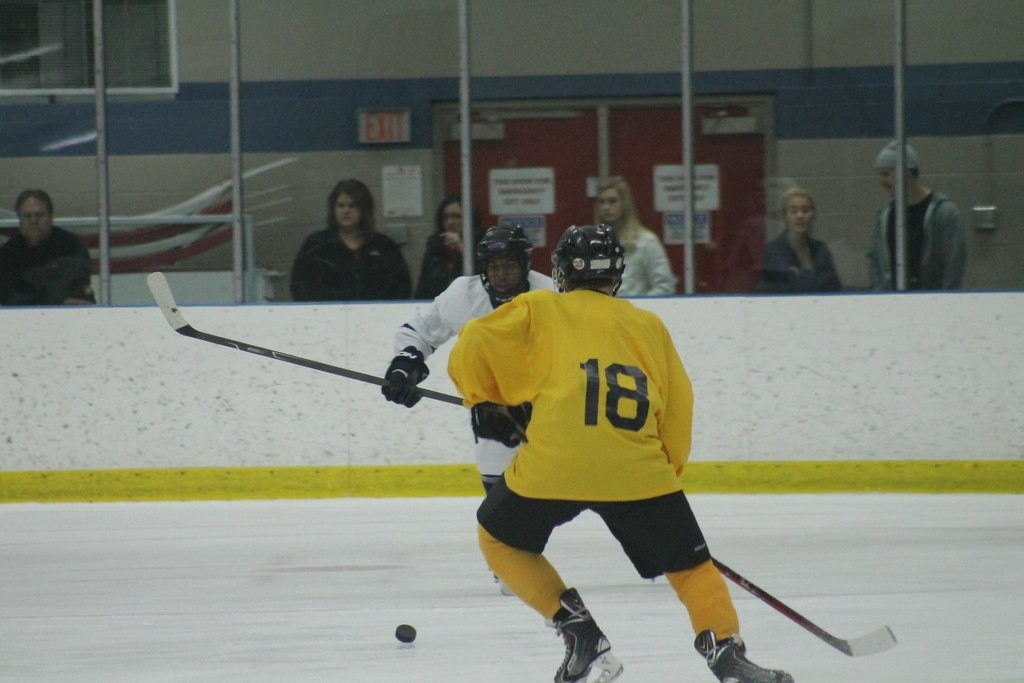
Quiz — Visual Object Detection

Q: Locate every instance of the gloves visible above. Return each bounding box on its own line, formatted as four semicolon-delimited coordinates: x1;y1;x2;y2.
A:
380;346;430;408
472;400;532;448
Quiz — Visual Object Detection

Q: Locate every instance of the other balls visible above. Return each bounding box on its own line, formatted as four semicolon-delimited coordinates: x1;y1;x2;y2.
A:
394;624;417;643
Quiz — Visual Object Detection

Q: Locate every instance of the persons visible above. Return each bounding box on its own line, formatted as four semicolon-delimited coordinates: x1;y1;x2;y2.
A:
385;218;564;595
0;188;96;307
288;139;969;305
450;223;796;683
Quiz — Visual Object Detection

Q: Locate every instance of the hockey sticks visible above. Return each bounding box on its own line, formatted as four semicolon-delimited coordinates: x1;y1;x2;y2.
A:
145;270;464;406
708;554;901;658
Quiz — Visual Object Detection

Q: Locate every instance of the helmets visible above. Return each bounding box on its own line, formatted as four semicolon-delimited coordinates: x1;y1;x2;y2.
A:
475;224;535;303
551;223;626;296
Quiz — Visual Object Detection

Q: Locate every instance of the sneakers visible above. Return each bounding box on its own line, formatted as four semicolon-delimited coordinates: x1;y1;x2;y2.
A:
545;588;623;682
694;629;795;682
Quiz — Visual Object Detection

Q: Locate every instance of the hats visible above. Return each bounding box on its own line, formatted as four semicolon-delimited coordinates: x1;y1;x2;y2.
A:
873;140;923;170
